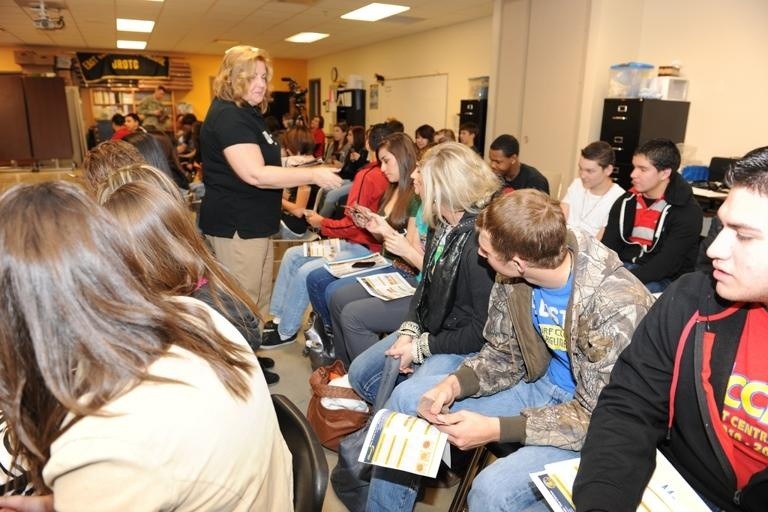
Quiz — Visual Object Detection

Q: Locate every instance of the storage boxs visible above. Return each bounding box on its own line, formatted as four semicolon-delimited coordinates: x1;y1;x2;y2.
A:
607;62;655;98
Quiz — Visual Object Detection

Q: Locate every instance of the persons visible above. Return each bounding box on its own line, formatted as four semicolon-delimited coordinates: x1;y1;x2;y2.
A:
0;113;295;511
137;87;167;130
262;115;768;512
200;44;344;386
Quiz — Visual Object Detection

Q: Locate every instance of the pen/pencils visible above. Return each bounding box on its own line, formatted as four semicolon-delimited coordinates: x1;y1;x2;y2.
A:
554;183;563;201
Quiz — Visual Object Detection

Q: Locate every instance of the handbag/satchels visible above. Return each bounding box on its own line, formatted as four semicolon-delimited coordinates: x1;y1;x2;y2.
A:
303;310;336;370
330;425;370;511
308;360;373;453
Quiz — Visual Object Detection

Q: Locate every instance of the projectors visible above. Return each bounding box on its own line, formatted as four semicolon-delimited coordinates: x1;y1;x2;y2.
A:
31;18;66;31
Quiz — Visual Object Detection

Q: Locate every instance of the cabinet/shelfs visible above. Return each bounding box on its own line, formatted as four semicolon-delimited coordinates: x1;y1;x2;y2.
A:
90;87;176;137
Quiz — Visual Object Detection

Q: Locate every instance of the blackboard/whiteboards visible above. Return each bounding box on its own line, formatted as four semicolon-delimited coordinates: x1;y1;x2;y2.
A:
366;73;450;143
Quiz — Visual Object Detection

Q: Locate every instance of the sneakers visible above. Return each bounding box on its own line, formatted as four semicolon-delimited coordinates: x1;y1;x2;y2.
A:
263;319;278;332
258;331;297;350
262;370;279;383
258;357;275;368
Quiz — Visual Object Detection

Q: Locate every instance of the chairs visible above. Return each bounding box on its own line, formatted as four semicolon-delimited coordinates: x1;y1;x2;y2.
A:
449;440;524;512
271;393;329;512
